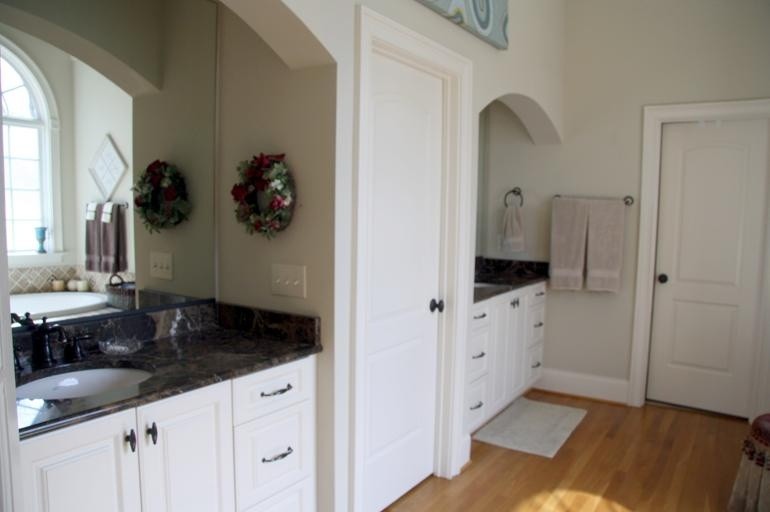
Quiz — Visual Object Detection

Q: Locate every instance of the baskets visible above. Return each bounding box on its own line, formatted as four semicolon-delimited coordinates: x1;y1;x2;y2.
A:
106;274;135;311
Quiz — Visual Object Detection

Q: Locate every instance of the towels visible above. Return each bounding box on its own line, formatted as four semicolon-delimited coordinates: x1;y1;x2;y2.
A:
500;199;526;252
549;197;625;294
85;202;127;273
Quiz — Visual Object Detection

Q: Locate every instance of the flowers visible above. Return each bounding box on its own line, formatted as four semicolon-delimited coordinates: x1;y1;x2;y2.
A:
128;159;192;234
229;151;296;241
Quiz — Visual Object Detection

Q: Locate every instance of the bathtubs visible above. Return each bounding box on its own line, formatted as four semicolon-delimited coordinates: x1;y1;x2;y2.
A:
9;290;110;317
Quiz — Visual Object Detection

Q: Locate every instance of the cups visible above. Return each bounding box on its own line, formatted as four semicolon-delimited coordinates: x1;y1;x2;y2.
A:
52;279;89;292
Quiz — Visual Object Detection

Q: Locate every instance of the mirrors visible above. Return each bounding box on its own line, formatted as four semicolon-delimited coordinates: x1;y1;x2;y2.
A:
0;0;219;331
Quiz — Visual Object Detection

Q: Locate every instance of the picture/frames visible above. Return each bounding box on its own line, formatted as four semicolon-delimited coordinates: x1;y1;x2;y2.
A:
84;132;128;203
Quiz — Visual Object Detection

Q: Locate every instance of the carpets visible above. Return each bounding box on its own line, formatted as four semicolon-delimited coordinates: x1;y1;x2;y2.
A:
473;396;588;459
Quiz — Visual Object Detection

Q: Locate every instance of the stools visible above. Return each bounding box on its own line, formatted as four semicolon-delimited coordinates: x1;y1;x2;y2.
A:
728;411;769;511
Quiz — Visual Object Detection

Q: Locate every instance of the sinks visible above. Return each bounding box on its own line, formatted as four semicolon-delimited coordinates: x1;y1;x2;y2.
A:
17;358;154;400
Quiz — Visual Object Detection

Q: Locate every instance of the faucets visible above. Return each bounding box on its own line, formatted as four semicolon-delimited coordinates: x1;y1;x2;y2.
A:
11;311;33;325
31;316;70;366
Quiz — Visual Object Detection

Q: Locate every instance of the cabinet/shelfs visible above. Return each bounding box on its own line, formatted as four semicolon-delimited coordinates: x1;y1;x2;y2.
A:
233;352;318;512
490;286;525;419
468;299;491;436
525;280;548;389
21;380;237;512
